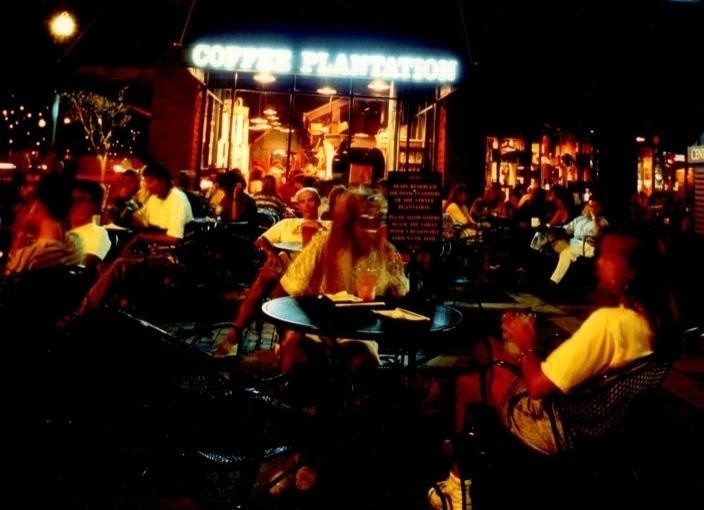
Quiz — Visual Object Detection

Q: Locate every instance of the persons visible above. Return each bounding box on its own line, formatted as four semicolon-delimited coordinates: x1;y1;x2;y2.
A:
0;144;704;509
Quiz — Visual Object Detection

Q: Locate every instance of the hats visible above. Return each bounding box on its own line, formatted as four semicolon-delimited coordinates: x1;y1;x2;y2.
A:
217;168;247;190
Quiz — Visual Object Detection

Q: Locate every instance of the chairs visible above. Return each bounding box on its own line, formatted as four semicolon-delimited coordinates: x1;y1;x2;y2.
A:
118;208;274;355
464;325;703;508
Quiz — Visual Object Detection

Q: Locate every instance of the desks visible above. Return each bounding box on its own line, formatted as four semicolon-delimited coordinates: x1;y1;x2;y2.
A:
262;292;462;414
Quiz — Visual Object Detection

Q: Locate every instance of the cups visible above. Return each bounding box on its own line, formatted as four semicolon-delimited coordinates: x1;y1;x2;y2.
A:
504;310;533;354
301;223;313;244
356;269;380;302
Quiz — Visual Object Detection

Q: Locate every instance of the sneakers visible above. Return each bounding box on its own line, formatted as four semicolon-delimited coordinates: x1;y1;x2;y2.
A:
427;472;473;510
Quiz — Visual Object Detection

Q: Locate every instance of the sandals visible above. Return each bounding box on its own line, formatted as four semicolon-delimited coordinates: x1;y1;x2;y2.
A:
270;466;317;496
